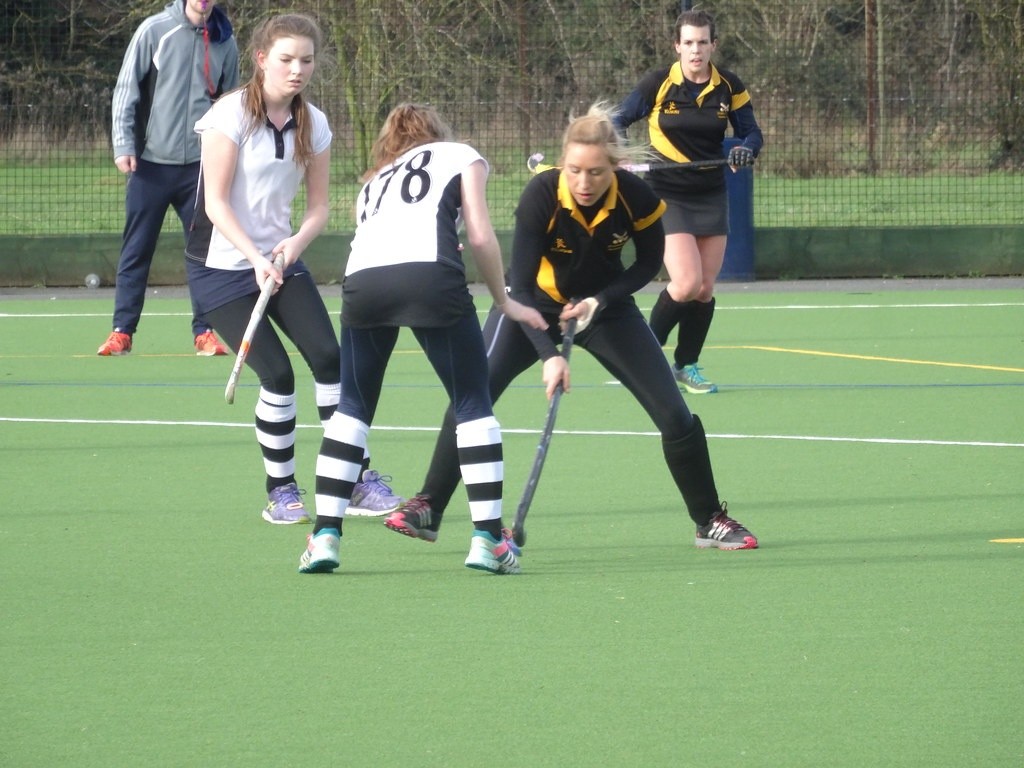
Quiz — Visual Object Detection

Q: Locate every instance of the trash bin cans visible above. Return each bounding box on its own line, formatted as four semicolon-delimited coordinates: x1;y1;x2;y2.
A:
716;136;755;282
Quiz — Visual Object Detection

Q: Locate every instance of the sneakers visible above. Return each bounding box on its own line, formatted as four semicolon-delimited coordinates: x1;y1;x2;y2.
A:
696;507;759;550
382;496;443;541
464;527;520;573
673;363;718;393
344;470;404;516
97;332;133;355
297;528;341;573
262;482;312;524
194;330;228;356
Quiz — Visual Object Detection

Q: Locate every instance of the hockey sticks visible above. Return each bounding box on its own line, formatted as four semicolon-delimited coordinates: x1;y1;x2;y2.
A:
526;151;756;182
224;251;284;406
510;294;582;548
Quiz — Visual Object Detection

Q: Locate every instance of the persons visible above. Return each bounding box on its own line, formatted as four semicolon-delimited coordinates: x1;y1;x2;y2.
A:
96;0;763;574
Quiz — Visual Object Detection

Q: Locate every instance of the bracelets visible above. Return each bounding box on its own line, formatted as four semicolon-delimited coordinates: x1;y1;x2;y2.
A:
494;297;510;308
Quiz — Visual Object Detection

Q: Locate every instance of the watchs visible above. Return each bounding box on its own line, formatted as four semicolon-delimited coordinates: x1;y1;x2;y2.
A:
595;294;608;311
